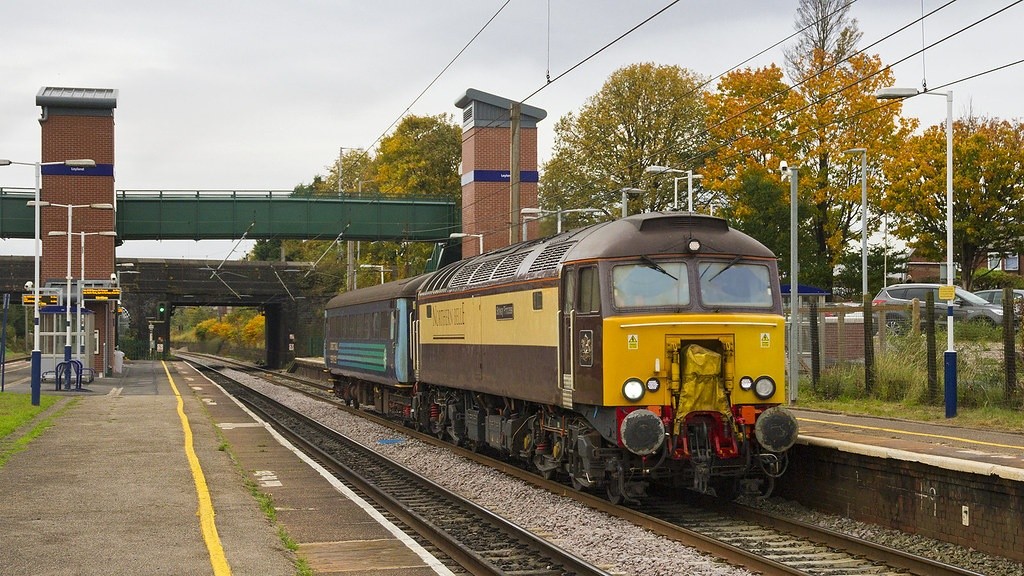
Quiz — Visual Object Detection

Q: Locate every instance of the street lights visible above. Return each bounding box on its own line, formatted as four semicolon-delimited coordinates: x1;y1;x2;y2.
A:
358;264;384;283
26;200;114;388
620;187;647;218
644;165;693;214
875;88;957;421
47;230;117;352
0;158;97;406
522;206;603;234
843;147;868;302
450;232;483;254
674;174;704;210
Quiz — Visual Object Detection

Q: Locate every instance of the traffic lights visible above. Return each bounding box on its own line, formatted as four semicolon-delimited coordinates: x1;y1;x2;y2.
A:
157;301;166;321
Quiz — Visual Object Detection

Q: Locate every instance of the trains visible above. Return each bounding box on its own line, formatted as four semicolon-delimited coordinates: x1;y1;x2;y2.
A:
322;212;801;504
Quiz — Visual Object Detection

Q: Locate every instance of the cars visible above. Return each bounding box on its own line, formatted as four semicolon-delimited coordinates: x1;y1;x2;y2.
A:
868;283;1019;336
956;289;1024;320
